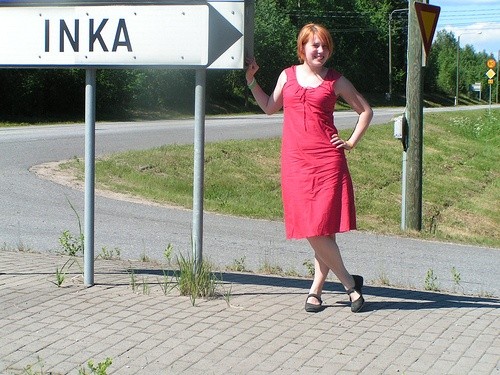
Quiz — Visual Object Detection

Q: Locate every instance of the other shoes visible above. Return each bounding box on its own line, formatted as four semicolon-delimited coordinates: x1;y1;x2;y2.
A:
347;275;364;312
305;294;322;312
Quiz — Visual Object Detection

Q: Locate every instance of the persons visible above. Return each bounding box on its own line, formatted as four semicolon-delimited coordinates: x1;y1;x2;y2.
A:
245;23;373;313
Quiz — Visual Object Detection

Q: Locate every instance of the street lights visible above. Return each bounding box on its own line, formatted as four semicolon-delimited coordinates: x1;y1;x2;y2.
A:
456;31;482;105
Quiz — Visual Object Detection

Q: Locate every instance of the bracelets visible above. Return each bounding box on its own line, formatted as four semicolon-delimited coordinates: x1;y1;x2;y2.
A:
345;141;353;153
247;77;256;89
